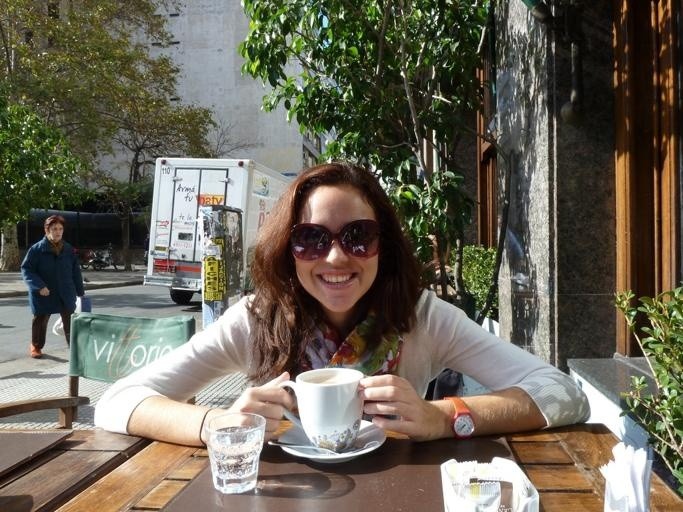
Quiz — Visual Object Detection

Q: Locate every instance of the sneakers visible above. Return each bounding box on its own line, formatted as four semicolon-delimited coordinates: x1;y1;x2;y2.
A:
30;342;42;358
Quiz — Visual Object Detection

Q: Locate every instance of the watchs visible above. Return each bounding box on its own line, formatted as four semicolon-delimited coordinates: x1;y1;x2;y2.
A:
444;395;477;439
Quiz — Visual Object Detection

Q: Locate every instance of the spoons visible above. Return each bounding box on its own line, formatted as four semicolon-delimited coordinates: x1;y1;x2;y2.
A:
264;436;379;455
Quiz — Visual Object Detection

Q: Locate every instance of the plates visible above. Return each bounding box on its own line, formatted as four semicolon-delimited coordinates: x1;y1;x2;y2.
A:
276;419;385;465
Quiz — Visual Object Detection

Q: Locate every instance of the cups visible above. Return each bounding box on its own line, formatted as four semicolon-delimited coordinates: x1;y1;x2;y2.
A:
273;368;367;449
204;412;266;492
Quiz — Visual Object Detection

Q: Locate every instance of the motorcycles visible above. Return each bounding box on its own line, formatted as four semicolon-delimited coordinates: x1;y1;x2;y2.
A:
69;244;117;271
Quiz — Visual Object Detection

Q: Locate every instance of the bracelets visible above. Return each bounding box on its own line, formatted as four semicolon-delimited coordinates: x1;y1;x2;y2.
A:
199;407;216;447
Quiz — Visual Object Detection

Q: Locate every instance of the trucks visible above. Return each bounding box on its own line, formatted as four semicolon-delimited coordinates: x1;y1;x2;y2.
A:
143;156;294;304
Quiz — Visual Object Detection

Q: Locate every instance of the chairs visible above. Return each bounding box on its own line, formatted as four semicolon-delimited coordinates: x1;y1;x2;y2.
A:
0;311;199;437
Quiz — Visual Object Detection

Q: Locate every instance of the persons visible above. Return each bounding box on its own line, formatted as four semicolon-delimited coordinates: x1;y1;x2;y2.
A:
21;216;85;358
94;163;590;450
143;234;150;266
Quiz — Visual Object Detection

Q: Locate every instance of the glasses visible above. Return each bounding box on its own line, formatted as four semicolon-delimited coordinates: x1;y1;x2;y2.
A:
288;217;383;260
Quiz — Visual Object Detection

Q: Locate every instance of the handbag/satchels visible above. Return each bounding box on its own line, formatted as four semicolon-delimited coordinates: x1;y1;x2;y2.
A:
52;317;64;337
